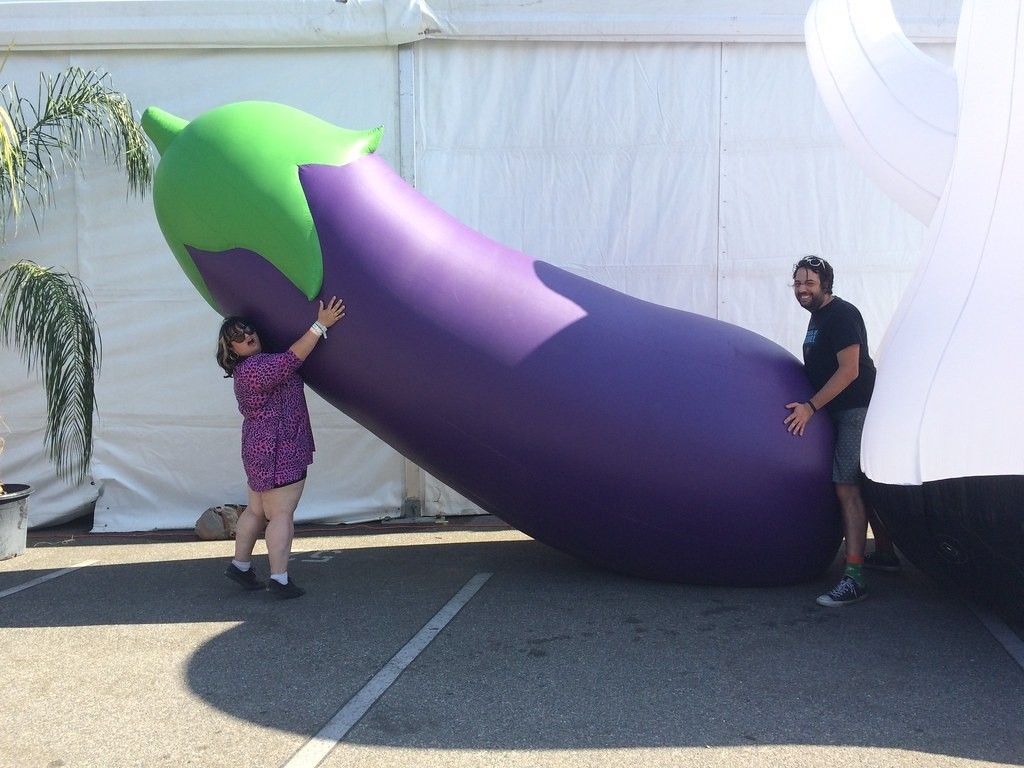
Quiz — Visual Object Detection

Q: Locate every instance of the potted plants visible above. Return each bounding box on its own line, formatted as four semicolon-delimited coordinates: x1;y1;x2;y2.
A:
0;67;156;562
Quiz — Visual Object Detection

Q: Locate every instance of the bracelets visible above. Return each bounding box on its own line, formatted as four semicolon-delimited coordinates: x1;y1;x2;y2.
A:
310;321;328;339
807;400;816;413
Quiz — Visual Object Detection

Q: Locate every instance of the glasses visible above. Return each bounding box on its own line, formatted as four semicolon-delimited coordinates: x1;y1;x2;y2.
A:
230;325;255;343
798;255;830;276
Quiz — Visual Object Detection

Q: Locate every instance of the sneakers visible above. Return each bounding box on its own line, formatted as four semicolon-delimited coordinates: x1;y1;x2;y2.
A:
267;579;305;598
816;575;871;608
863;550;900;572
224;563;263;591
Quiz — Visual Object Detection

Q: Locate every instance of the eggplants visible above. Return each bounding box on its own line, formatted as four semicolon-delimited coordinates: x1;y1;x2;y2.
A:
139;100;846;583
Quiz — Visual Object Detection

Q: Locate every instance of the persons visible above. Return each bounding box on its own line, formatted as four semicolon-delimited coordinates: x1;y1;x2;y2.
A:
784;256;877;607
216;296;346;599
